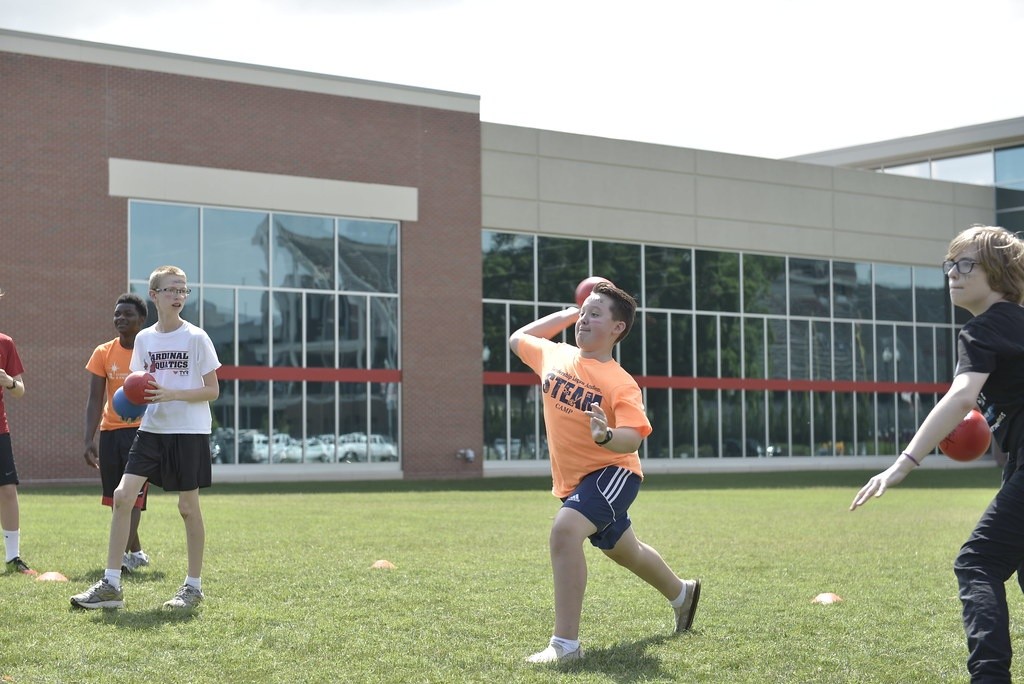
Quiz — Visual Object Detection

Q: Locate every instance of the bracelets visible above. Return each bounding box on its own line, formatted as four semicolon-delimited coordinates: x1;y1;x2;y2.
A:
901;450;921;467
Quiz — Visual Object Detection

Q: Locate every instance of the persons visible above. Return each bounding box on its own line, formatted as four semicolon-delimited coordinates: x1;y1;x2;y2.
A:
69;265;222;610
84;293;150;574
850;226;1024;684
0;333;36;574
509;285;701;665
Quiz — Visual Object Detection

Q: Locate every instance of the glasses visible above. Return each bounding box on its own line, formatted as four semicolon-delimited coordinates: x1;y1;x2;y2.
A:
152;287;192;297
943;258;982;275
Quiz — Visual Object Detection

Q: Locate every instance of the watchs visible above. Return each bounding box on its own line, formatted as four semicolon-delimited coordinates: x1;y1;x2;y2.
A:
595;428;612;445
6;379;16;389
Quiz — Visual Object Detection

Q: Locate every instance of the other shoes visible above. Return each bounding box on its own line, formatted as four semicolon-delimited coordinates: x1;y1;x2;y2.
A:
524;643;581;666
672;578;700;632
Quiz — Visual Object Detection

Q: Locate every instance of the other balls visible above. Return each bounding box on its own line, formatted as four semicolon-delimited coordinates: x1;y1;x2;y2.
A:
937;409;992;461
577;272;614;309
110;386;148;420
122;368;157;405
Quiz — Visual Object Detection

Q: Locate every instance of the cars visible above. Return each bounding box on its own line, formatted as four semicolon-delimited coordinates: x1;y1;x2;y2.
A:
717;437;781;459
239;430;397;463
494;437;549;459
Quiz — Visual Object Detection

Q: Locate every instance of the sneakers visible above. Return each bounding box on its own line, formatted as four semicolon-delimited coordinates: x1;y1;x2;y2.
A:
164;584;202;609
70;579;123;608
6;557;38;575
130;552;149;566
121;553;131;574
